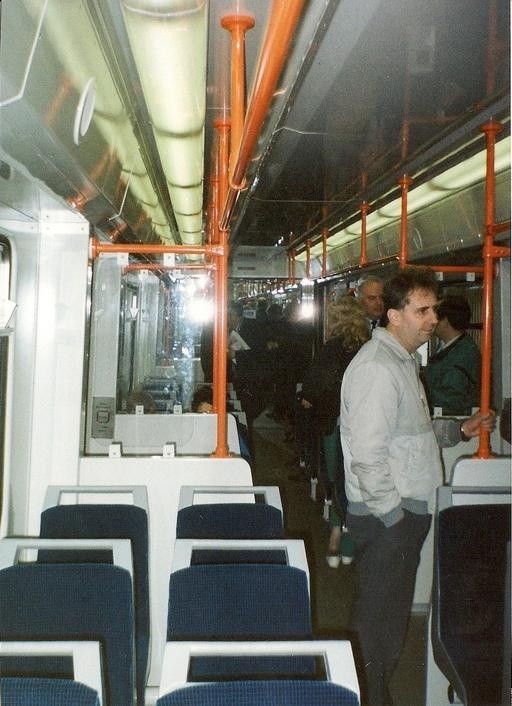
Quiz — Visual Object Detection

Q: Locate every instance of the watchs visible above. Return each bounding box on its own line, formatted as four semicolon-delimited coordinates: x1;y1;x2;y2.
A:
460;426;472;441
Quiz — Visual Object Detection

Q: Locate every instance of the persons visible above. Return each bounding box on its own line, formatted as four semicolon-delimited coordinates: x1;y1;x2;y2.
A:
422;295;480;416
304;294;373;567
200;293;316;384
127;393;159;415
192;387;254;467
342;268;494;706
354;274;385;334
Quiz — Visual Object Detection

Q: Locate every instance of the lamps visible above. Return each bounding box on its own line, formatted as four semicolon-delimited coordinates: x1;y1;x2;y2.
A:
430;137;511;193
295;251;320;263
174;212;203;233
121;4;208;137
380;183;460;219
326;228;360;247
345;212;400;236
307;242;336;257
154;128;205;187
180;232;204;260
167;181;203;215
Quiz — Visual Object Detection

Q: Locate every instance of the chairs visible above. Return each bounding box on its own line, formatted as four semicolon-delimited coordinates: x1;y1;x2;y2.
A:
176;484;288;564
156;642;359;706
36;486;153;682
164;537;315;679
0;641;105;706
142;363;246;429
0;536;136;706
430;486;511;706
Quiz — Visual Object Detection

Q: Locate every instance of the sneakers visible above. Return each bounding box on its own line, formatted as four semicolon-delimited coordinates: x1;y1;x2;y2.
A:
342;553;354;566
326;553;341;569
